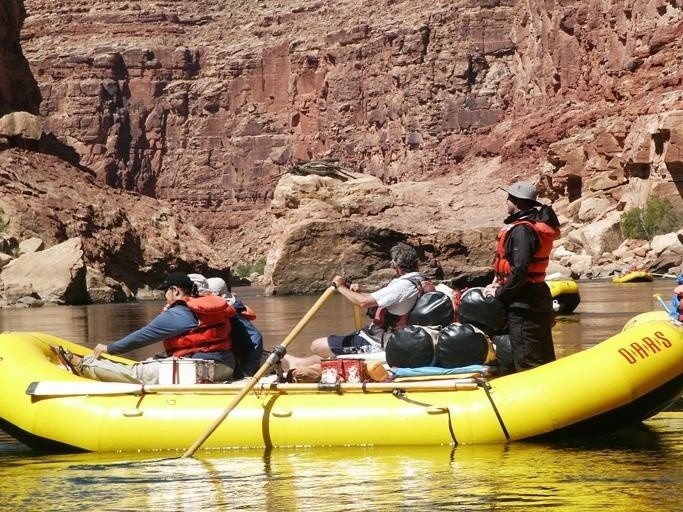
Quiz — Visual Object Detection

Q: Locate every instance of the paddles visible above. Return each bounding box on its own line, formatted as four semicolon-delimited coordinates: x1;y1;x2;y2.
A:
26;382;479;397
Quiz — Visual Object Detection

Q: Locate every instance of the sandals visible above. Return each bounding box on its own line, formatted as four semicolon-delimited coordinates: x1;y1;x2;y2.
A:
56;344;83;377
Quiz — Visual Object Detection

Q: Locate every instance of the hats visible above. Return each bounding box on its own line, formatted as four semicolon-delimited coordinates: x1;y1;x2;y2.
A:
498;180;544;207
157;271;235;305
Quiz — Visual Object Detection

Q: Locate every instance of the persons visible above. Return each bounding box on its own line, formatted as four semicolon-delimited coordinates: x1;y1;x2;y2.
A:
48;273;263;385
484;180;560;373
280;243;436;372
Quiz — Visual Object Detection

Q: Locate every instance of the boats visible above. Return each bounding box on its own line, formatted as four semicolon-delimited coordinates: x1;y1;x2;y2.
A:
612;271;653;284
544;280;581;314
